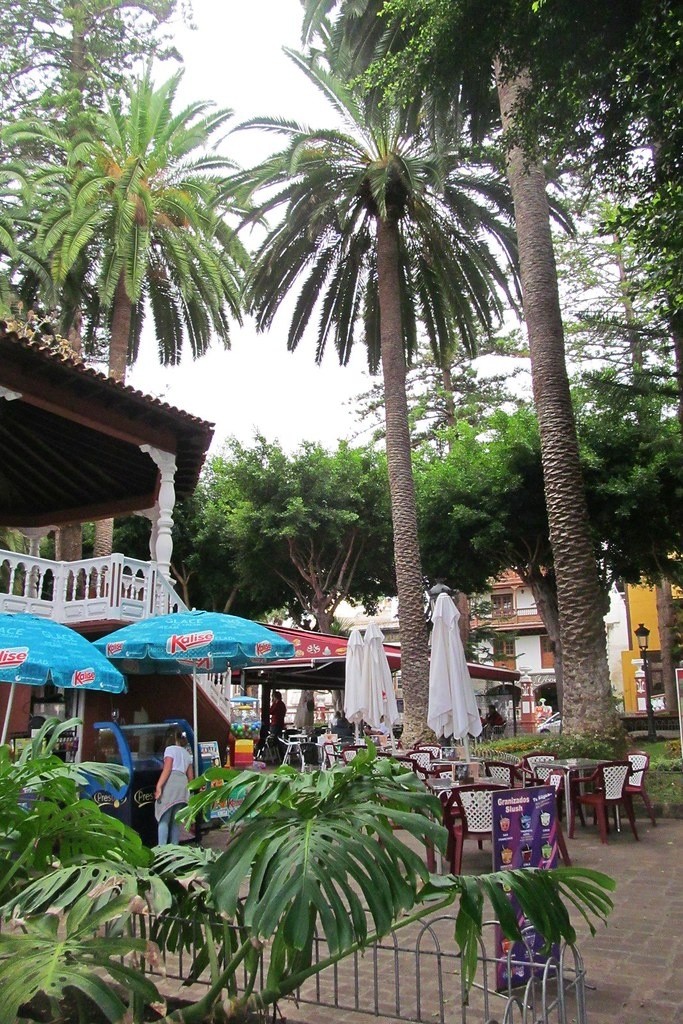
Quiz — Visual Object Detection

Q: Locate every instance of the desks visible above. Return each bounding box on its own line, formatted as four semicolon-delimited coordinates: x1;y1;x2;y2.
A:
532;759;619;839
423;777;510;874
431;758;484;781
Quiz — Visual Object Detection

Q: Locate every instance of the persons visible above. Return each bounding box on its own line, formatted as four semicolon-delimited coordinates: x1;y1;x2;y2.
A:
477;704;503;734
269;691;390;739
155;733;193;846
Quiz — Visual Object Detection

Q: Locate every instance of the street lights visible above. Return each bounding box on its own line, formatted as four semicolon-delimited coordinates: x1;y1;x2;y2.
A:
634;623;657;738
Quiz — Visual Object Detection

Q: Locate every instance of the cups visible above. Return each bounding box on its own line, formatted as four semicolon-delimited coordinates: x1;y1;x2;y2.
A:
499;813;553;977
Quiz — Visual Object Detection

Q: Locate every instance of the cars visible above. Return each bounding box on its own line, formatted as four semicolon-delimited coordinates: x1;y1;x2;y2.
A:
536;711;561;736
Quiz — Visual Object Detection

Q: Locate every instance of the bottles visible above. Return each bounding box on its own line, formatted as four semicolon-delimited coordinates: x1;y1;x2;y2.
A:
55;737;78;751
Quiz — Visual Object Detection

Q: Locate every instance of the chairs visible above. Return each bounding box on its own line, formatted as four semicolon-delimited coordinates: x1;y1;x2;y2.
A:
491;721;506;740
279;735;658;879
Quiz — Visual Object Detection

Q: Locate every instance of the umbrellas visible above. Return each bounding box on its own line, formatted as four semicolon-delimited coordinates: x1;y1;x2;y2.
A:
344;621;399;752
427;593;482;764
92;607;295;780
0;611;128;747
